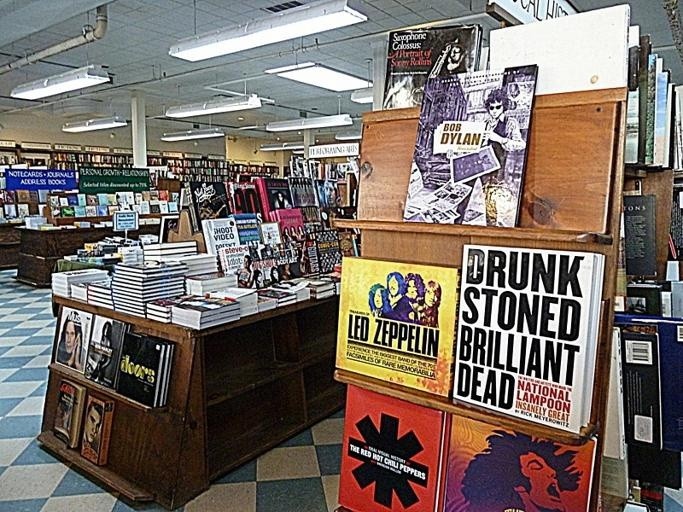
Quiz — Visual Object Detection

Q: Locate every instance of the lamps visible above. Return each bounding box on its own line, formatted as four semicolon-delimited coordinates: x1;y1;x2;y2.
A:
9;46;116;102
61;116;129;134
158;0;374;154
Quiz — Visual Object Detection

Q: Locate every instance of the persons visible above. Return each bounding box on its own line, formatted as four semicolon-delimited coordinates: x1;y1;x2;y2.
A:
369;273;442;328
462;428;583;512
84;401;102;443
483;87;525;181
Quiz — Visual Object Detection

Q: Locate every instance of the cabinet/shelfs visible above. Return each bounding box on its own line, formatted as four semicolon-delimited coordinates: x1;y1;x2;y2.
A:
0;140;350;511
329;88;632;511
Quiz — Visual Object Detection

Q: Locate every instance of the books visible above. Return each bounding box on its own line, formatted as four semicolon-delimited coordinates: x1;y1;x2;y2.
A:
338;385;449;512
453;245;605;433
335;256;462;398
604;24;683;498
51;208;356;407
444;415;598;512
383;24;482;110
402;64;539;227
80;393;115;466
0;155;357;232
53;380;86;449
